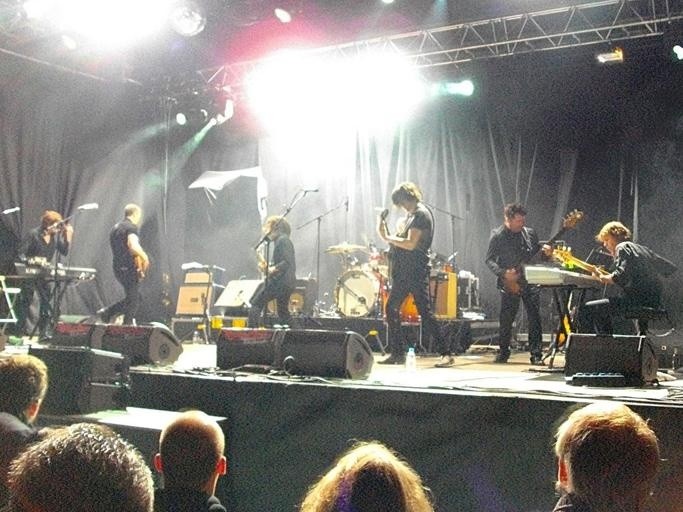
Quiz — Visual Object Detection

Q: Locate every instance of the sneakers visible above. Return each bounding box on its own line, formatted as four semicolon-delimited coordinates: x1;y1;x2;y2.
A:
376;355;406;365
493;352;512;364
434;358;455;369
96;308;108;324
529;354;545;365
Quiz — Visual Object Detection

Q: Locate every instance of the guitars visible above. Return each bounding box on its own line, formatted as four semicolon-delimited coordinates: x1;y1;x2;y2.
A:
497;209;584;297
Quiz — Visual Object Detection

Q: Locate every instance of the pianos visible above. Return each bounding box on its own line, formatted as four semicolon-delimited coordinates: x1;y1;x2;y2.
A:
524;264;601;289
0;260;96;284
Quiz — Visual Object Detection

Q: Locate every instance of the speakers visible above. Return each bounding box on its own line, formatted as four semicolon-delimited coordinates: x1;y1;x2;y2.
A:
566;332;659;385
277;329;376;380
50;321;107;350
102;324;183;366
176;283;225;316
423;317;469;352
216;327;288;371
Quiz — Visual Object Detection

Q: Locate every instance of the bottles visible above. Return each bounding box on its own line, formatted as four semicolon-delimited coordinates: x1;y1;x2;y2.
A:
192;330;200;345
671;347;681;370
405;347;417;379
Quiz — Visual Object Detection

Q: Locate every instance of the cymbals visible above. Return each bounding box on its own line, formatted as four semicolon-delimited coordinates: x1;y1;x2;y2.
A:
325;246;369;252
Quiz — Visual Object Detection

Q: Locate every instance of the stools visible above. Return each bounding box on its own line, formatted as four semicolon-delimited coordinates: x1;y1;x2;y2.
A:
627;304;668;335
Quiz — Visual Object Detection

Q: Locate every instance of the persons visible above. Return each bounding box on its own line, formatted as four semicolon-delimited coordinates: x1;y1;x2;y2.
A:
1;354;49;509
301;442;434;512
550;398;661;512
485;204;553;366
8;421;154;512
578;221;677;332
15;210;74;342
95;204;148;326
151;412;230;511
377;184;454;369
249;216;302;327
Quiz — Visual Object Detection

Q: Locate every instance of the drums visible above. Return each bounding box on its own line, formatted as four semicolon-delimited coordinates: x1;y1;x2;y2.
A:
335;264;388;318
381;284;419;323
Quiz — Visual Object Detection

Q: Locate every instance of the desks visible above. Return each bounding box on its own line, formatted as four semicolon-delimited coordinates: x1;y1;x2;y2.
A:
42;405;229;435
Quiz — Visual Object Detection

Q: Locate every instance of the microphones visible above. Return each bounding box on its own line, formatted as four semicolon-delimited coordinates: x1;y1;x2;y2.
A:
3;206;20;215
345;199;349;212
77;203;98;210
303;188;319;193
